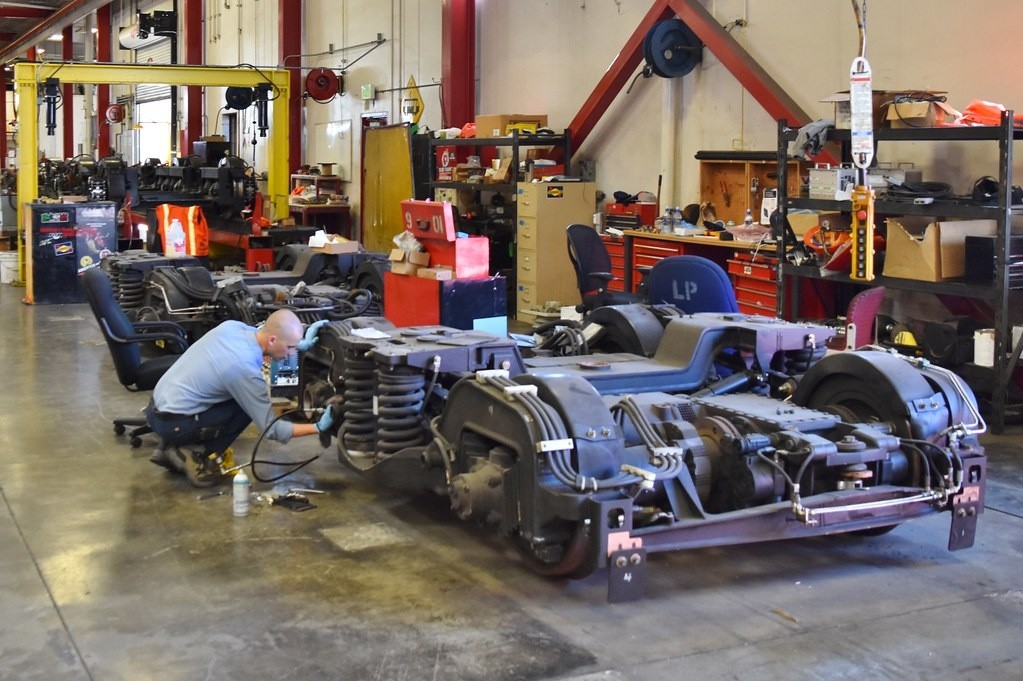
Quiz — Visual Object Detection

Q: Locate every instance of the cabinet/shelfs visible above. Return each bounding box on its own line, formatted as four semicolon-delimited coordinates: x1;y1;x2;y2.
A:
516;182;596;324
424;128;571;317
779;108;1022;433
25;200;118;304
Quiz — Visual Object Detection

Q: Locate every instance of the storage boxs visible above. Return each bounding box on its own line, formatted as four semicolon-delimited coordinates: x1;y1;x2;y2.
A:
416;268;456;280
389;249;430;276
474;114;548;139
310;235;359;254
787;213;849;241
886;100;963;128
881;216;998;285
399;199;489;280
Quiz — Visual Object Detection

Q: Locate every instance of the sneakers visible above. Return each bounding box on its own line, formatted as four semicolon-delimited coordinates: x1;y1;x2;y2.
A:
164;443;215;487
149;448;177;472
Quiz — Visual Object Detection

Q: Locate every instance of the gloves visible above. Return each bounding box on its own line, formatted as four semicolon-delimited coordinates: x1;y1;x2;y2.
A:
298;320;329;351
314;404;334;433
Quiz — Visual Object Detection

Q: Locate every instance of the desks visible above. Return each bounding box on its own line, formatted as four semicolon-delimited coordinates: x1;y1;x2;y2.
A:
289;204;352;244
599;227;846;349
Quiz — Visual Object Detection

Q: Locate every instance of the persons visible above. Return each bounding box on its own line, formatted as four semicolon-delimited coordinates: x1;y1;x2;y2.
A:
145;308;335;489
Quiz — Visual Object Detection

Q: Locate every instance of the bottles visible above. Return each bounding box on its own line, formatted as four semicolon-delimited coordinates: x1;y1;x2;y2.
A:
673;206;682;231
662;206;671;233
233;468;249;517
743;208;753;228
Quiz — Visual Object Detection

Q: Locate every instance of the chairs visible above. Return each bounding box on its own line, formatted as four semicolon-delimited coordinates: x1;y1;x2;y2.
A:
563;224;651;317
646;256;740;362
82;265;191;450
